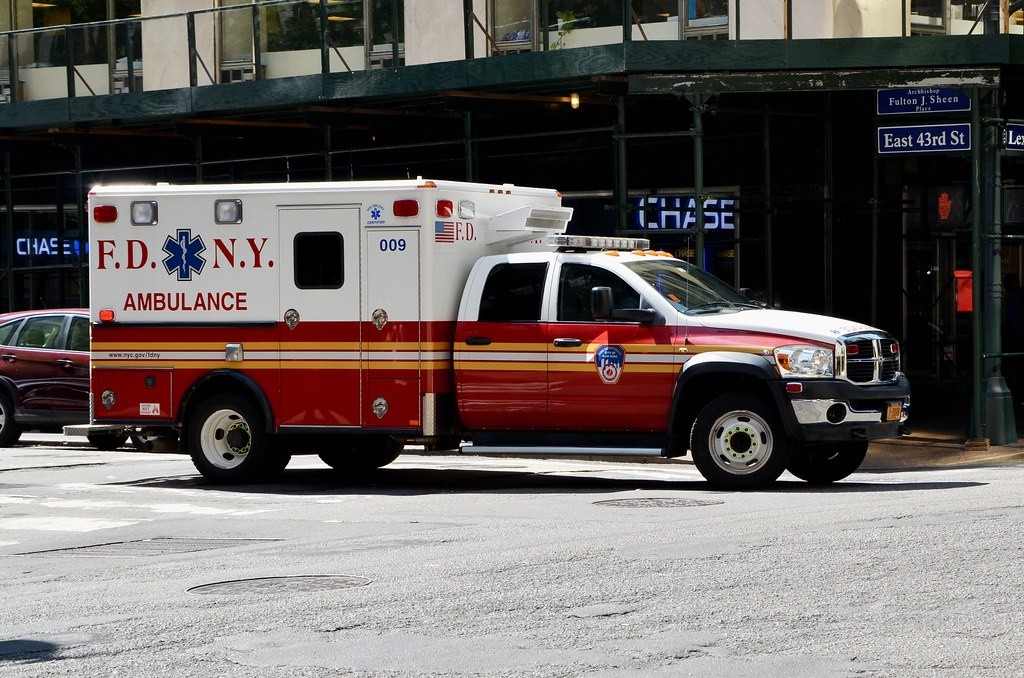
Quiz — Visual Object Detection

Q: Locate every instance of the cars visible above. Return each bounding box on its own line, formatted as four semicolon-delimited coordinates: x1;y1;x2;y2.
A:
0;309;183;452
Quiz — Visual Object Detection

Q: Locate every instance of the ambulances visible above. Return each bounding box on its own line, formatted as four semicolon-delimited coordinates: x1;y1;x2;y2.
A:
63;176;916;491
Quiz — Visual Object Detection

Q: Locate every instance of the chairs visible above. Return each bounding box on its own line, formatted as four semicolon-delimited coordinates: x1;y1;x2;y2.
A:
21;329;45;347
561;276;593;321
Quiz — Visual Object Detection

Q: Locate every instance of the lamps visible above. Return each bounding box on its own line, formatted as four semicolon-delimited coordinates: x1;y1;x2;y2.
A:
571;93;580;109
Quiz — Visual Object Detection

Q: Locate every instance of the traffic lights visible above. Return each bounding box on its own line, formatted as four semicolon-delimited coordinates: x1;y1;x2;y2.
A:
926;178;970;233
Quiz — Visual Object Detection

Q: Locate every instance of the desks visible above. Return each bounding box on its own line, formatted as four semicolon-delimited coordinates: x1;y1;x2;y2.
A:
685;16;729;40
911;22;947;36
369;48;406;68
219;59;266;83
0;77;25;104
112;68;143;93
492;39;544;55
